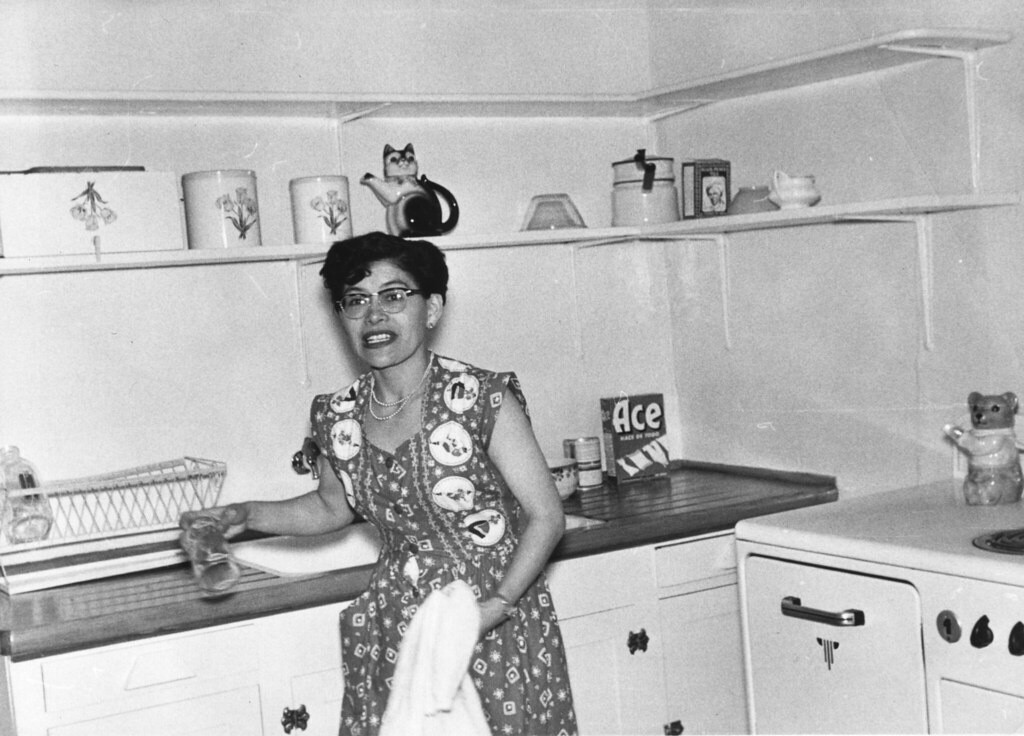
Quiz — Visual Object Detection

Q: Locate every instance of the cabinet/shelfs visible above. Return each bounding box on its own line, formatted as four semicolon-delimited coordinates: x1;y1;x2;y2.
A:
0;26;1018;352
546;528;750;736
0;601;352;736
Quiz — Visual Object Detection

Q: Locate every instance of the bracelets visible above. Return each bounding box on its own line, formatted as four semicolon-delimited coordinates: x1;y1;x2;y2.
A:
492;592;518;611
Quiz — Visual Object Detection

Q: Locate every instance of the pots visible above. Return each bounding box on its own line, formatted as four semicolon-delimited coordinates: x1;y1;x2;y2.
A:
610;147;680;228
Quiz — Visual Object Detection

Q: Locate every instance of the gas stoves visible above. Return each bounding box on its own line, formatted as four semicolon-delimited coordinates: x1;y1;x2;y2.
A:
935;515;1024;699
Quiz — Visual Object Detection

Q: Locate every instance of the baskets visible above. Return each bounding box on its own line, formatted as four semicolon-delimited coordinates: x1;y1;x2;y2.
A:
0;457;225;540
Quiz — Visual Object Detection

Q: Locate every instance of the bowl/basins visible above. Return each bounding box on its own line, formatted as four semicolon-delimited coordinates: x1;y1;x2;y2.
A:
545;456;578;501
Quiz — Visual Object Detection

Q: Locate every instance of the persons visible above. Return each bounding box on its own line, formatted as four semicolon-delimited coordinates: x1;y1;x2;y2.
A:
180;229;586;736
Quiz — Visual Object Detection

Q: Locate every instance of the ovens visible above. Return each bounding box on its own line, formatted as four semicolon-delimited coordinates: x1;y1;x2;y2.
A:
727;539;932;734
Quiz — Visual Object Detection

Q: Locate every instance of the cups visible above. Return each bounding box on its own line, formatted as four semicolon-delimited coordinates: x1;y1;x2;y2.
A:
181;169;262;249
182;516;241;593
288;175;354;244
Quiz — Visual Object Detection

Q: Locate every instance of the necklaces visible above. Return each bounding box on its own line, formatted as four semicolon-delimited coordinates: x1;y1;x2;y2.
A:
369;347;436;420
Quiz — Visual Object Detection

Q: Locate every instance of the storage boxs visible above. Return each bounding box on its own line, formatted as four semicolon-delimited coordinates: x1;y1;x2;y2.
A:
681;158;730;220
600;392;672;485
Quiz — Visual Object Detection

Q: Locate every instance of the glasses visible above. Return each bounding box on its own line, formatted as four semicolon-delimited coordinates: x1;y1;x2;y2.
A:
334;288;426;319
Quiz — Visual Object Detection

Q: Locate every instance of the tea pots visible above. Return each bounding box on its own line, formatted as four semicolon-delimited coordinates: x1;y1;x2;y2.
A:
768;170;821;210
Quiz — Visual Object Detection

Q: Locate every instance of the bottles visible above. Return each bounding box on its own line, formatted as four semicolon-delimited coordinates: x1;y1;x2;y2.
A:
0;445;54;545
